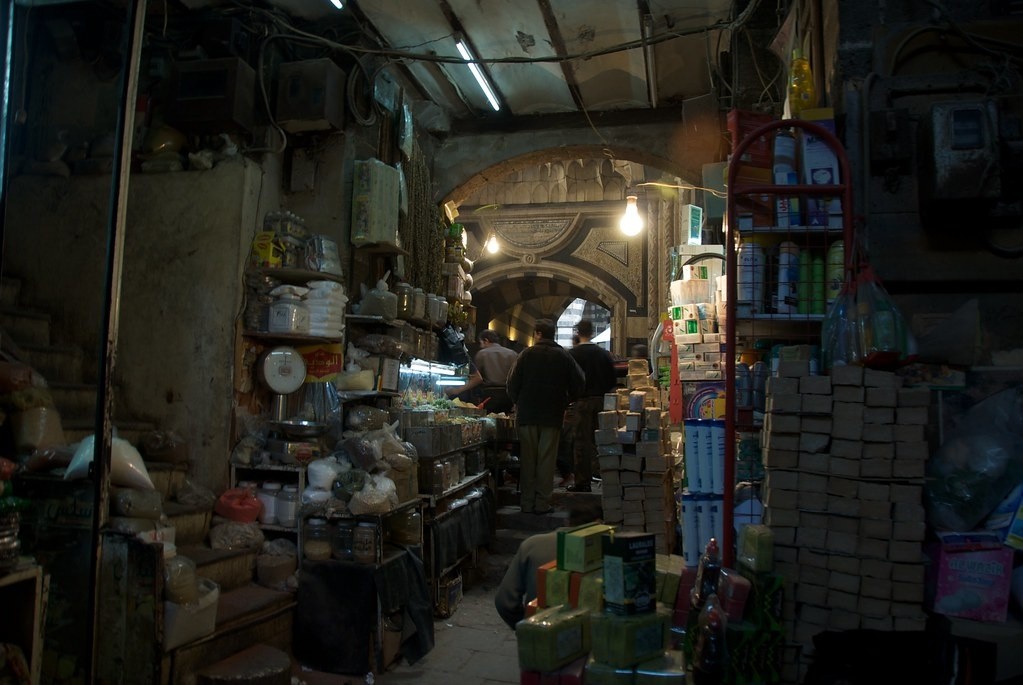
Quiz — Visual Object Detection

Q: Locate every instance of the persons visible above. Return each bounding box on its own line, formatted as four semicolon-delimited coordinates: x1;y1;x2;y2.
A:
447;349;483;403
567;319;615;492
556;412;575;487
505;318;586;515
494;506;595;630
571;335;580;346
474;329;518;416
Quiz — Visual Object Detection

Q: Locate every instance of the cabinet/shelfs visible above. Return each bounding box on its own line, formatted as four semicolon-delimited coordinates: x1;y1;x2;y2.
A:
231;239;490;685
720;120;852;565
0;564;42;685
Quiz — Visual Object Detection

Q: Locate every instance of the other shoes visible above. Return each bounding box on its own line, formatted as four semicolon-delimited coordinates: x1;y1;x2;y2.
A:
533;506;554;515
566;484;592;493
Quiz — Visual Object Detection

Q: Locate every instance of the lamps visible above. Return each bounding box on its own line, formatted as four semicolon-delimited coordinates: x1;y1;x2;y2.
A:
450;30;506;115
325;0;347;15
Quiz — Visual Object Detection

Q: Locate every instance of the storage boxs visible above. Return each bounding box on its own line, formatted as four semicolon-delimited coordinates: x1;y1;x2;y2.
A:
448;276;466;303
436;574;463;620
162;579;220;651
515;203;928;685
443;260;465;280
925;546;1013;624
368;358;400;391
405;425;462;456
253;231;284;270
702;162;732;224
388;464;418;502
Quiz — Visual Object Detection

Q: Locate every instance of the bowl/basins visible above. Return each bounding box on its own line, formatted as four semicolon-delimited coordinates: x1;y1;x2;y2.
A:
266;420;331;438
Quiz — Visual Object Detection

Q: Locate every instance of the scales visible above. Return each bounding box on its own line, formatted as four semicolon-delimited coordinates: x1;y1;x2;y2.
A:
256;344;330;464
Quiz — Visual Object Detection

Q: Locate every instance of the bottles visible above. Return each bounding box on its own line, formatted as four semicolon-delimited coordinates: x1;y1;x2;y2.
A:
387;320;439;361
264;211;309;267
305;518;380;563
238;481;299;527
390;282;449;325
687;534;728;674
392;508;421;549
267;293;309;335
418;445;486;495
790;48;816;109
